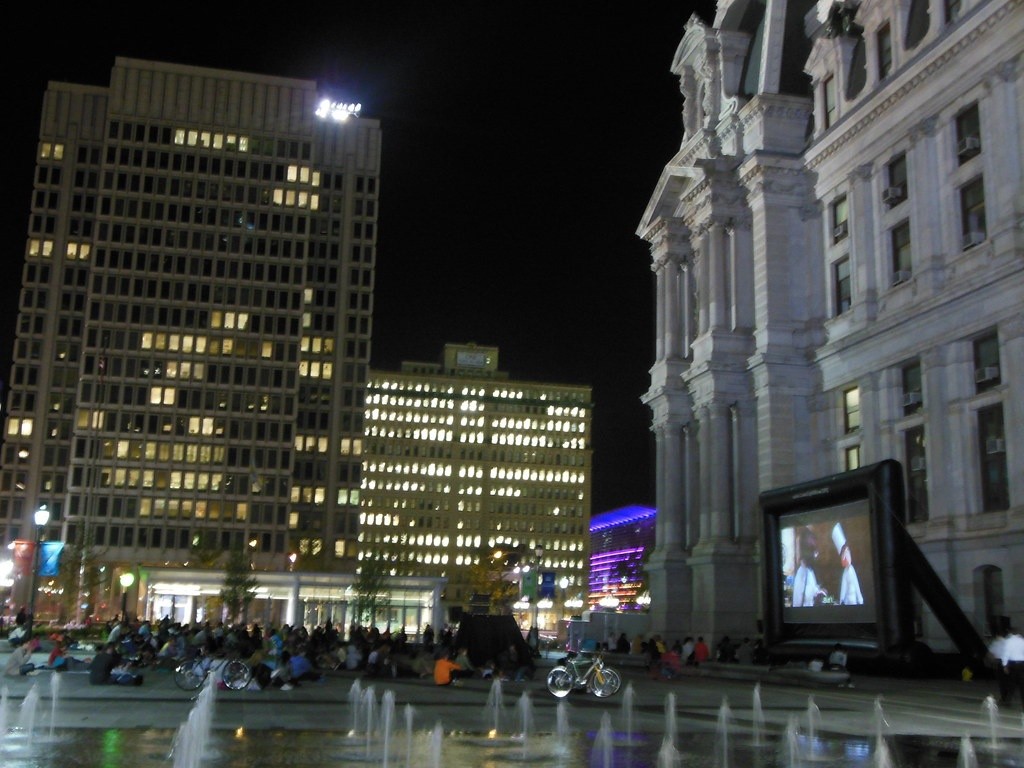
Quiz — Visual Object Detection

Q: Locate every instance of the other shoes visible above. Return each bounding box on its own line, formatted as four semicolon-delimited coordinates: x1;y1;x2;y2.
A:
848;684;855;688
839;683;845;688
279;683;294;691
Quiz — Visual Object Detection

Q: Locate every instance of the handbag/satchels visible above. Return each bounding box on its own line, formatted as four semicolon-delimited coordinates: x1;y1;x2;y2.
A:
246;679;261;691
688;650;695;661
809;659;824;673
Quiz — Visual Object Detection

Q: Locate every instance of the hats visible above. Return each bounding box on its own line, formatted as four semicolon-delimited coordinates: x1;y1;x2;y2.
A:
831;523;847;558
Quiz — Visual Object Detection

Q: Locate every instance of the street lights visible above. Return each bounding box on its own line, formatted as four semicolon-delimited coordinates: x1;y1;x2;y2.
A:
512;565;530;632
25;504;50;641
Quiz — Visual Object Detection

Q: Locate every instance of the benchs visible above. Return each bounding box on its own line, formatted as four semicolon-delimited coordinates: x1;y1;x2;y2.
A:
695;661;770;682
767;668;849;690
605;654;652;673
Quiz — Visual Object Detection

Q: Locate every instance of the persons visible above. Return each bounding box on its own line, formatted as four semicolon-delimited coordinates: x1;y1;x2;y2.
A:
435;649;461;684
832;522;864;604
9;626;27;645
793;531;830;608
32;633;41;651
49;642;82;672
109;663;142;685
88;641;121;686
100;614;541;688
605;631;752;678
16;608;27;624
6;639;35;676
831;643;857;688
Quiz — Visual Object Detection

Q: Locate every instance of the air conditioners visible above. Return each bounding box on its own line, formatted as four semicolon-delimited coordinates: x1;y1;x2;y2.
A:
910;457;926;472
882;187;902;204
975;367;999;383
957;137;980;156
986;439;1005;455
834;225;845;238
961;232;986;250
892;270;912;286
903;392;921;406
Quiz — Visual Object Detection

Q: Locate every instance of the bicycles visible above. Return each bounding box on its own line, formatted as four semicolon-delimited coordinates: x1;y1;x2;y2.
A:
547;648;621;698
175;642;252;691
